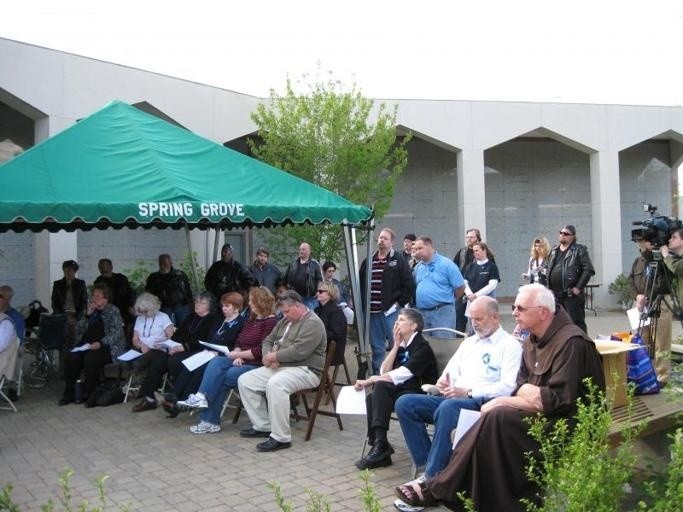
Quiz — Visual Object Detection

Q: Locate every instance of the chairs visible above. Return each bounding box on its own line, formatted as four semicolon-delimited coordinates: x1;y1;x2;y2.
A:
0;346;248;425
229;323;352;440
359;325;469;483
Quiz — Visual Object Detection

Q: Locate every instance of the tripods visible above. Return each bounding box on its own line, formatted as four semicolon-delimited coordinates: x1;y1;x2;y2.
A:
638;248;683;360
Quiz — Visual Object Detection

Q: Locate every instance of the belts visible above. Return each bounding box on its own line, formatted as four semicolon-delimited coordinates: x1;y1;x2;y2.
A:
419;302;450;311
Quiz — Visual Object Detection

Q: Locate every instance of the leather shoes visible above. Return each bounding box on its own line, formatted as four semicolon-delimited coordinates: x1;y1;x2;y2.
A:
59;380;291;450
356;440;394;470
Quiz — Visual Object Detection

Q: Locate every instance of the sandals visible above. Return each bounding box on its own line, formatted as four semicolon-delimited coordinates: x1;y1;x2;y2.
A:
396;482;444;506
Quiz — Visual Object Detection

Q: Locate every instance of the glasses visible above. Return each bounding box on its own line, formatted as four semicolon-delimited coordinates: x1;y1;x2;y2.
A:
512;305;543;312
560;231;574;236
315;289;327;293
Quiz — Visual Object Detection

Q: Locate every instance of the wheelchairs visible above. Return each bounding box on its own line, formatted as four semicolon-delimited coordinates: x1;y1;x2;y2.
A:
18;299;70;392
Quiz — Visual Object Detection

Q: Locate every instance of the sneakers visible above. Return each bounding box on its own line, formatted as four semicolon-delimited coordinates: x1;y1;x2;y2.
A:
394;474;429;511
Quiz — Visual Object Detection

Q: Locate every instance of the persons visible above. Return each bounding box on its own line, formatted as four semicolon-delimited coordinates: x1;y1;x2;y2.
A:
627;225;682;389
134;243;354;450
395;293;523;510
354;309;440;469
1;284;27;403
47;254;194;417
394;283;605;512
357;227;500;370
520;225;595;335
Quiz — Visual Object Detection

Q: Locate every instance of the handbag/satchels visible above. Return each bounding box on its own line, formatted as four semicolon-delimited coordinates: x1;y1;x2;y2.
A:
84;346;113;378
611;332;659;395
24;301;49;327
171;329;203;352
